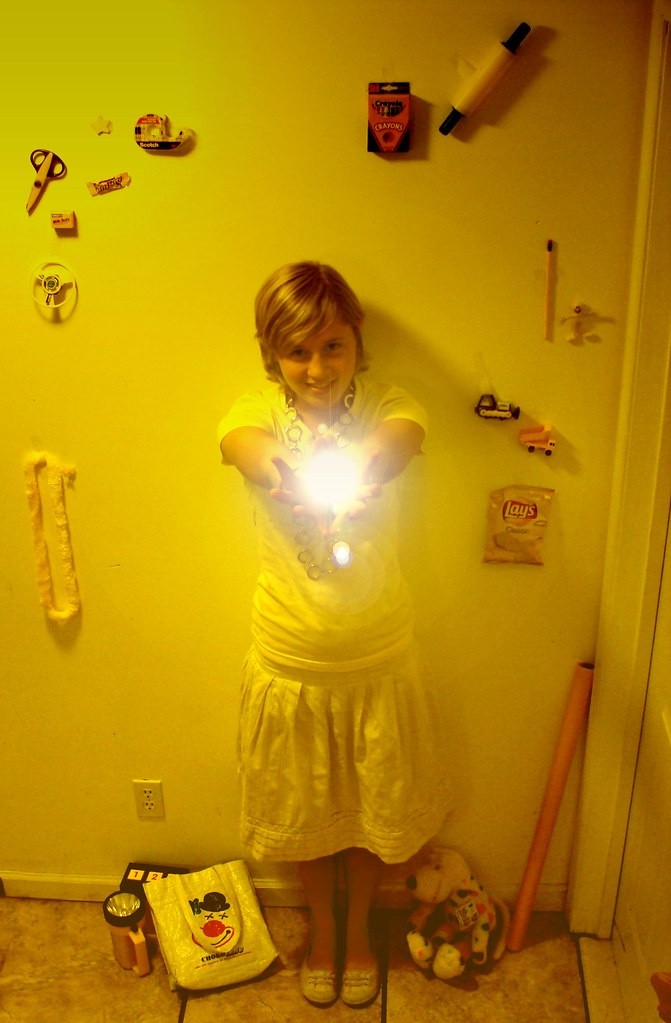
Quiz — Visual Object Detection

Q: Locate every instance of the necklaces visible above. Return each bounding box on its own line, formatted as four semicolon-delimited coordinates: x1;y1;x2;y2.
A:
285;385;354;581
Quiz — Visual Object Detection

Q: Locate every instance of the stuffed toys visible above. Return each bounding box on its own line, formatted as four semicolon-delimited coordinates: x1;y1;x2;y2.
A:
406;847;510;981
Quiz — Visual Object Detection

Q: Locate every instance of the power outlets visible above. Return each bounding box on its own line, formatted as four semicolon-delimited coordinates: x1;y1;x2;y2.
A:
132;779;165;817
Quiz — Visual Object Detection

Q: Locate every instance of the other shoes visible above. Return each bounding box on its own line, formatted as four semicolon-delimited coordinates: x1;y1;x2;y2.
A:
339;928;379;1004
300;929;336;1003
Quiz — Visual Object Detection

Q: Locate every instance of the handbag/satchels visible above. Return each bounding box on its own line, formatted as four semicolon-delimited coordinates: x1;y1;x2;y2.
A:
143;859;279;992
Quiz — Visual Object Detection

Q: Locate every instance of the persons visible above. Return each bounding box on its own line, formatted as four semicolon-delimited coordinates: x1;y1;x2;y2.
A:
217;260;429;1007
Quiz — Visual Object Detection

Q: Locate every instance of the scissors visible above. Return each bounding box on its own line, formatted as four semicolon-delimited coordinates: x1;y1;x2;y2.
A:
24;149;68;214
